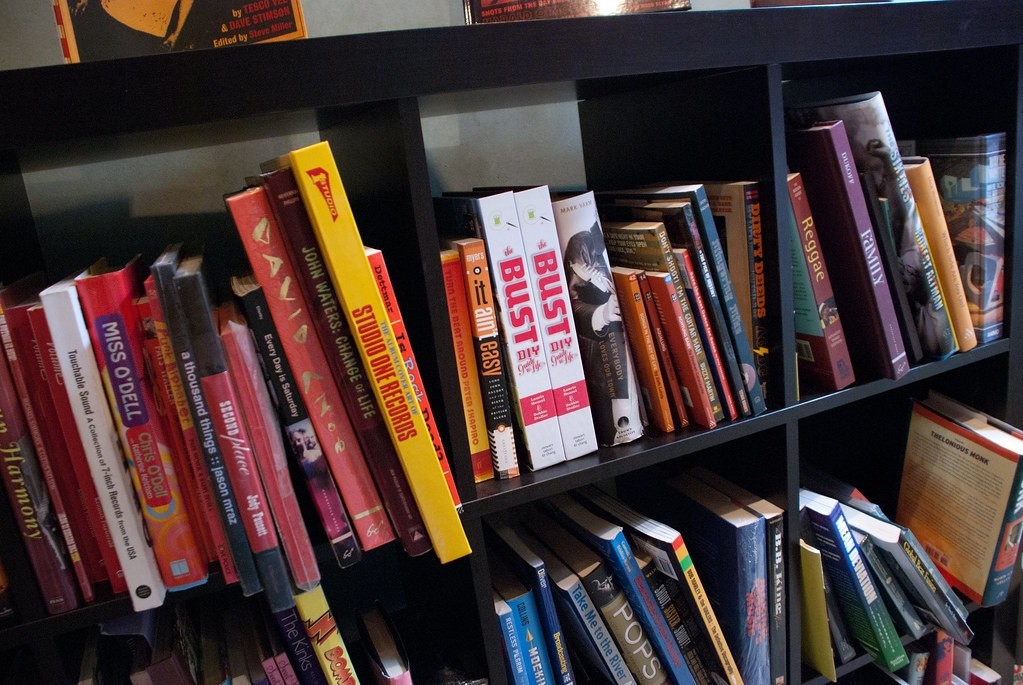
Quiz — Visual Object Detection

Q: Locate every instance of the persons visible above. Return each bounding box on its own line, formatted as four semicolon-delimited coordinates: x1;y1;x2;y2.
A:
900;246;940;360
289;428;321;464
563;206;629;447
819;302;840;328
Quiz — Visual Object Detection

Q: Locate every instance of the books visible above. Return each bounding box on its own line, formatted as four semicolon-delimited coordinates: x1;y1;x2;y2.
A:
1;88;1023;685
462;0;691;27
52;0;309;65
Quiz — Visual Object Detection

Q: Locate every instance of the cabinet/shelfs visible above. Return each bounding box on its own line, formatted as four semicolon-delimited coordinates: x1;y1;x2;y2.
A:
0;0;1023;685
